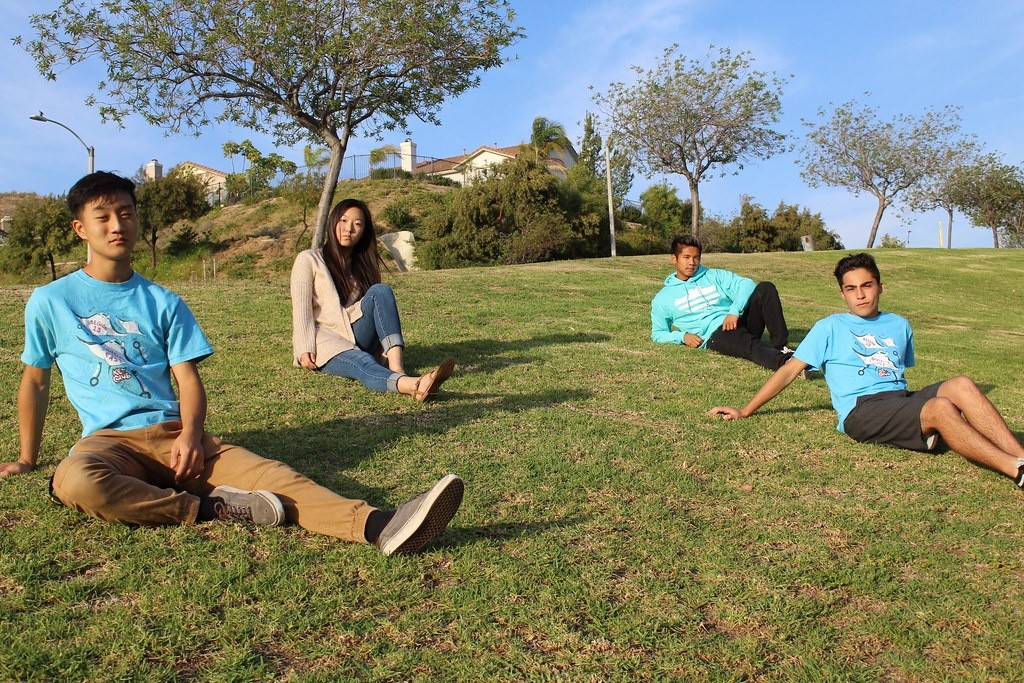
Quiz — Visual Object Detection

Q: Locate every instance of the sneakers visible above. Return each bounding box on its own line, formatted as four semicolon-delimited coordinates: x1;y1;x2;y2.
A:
207;485;285;528
376;473;464;557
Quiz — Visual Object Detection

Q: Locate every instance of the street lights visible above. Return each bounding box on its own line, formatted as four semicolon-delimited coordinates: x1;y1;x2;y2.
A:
604;118;636;258
28;110;98;268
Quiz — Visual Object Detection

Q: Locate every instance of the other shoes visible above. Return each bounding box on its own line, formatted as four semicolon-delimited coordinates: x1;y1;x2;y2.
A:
785;358;808;380
779;346;820;371
1013;457;1024;487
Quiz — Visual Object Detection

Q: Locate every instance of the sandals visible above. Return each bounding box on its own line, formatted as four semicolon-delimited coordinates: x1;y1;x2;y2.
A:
412;357;455;403
393;369;408;376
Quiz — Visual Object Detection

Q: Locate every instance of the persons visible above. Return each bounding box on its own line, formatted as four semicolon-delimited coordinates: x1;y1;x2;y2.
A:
702;251;1024;493
650;234;812;382
289;198;457;403
0;168;467;561
